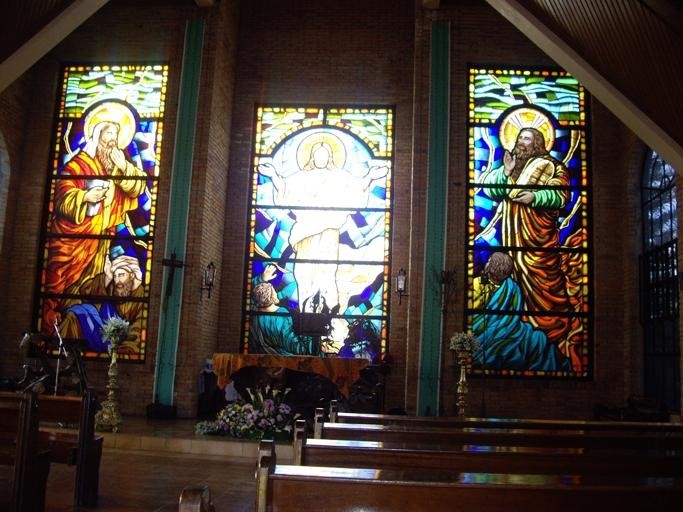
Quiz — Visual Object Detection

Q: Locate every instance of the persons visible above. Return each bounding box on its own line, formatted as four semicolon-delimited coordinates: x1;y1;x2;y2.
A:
469;127;572;371
250;141;388;360
39;121;144;355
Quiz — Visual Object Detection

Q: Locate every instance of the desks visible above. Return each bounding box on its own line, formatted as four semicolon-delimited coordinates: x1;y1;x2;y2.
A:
211;350;369;410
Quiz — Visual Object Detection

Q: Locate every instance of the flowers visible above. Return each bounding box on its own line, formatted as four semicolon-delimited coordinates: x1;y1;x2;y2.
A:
98;312;134;357
449;330;479;360
193;382;307;442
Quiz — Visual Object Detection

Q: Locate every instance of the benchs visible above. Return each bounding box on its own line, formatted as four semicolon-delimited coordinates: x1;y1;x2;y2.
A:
0;384;104;512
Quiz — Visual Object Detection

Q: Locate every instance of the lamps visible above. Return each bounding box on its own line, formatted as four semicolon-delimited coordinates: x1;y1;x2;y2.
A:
201;261;216;298
395;268;409;305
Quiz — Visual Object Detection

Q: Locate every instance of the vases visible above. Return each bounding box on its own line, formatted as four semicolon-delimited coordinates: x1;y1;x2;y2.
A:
456;351;469;394
107;336;120;377
